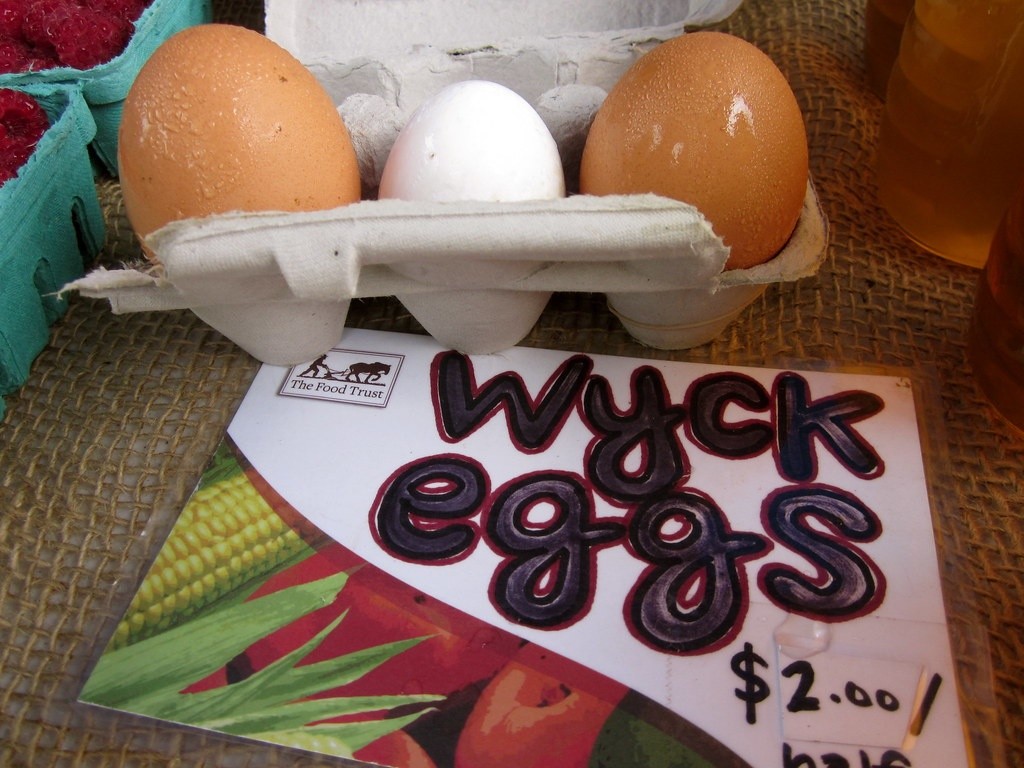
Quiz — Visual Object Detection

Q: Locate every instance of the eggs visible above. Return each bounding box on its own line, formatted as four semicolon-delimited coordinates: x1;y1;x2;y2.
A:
118;23;360;261
576;30;808;271
378;80;568;201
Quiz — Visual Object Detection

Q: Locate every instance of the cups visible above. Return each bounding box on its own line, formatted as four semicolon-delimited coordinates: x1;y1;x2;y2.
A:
862;0;1024;438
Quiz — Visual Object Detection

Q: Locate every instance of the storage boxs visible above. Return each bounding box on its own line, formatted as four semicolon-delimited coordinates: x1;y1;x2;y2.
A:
0;0;215;183
0;82;107;422
